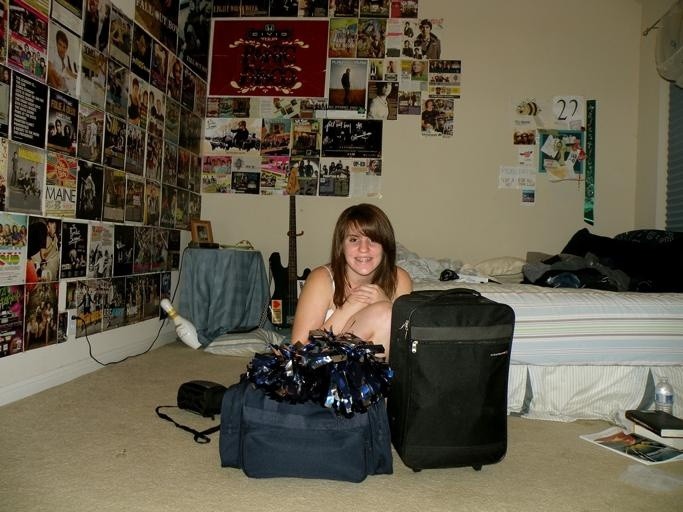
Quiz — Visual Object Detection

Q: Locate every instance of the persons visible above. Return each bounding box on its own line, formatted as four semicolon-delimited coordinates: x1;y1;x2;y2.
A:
289;199;418;362
1;1;456;348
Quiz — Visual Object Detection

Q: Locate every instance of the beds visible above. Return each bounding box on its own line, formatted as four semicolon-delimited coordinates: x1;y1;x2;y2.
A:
409;272;682;419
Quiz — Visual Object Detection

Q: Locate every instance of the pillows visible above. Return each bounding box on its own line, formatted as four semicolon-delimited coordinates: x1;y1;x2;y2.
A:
202;330;288;358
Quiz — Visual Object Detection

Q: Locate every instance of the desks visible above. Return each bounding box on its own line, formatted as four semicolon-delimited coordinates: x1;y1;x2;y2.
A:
184;248;263;332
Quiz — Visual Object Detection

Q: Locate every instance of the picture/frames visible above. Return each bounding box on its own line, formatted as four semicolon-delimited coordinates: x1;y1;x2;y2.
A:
191;219;212;244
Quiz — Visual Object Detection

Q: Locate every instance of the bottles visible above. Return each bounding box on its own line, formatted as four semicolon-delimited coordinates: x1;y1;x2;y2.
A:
654;376;674;417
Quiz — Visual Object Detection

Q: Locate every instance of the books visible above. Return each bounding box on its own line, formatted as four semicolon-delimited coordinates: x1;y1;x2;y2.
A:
578;425;683;466
627;409;683;439
633;427;683;454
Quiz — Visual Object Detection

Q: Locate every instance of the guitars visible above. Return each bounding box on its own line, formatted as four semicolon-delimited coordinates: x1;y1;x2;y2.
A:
268;167;311;329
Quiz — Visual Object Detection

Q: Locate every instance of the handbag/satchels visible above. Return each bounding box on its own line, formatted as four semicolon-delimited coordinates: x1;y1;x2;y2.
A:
219;375;394;484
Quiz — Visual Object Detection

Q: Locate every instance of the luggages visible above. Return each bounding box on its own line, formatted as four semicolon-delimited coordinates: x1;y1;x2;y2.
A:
385;288;514;473
